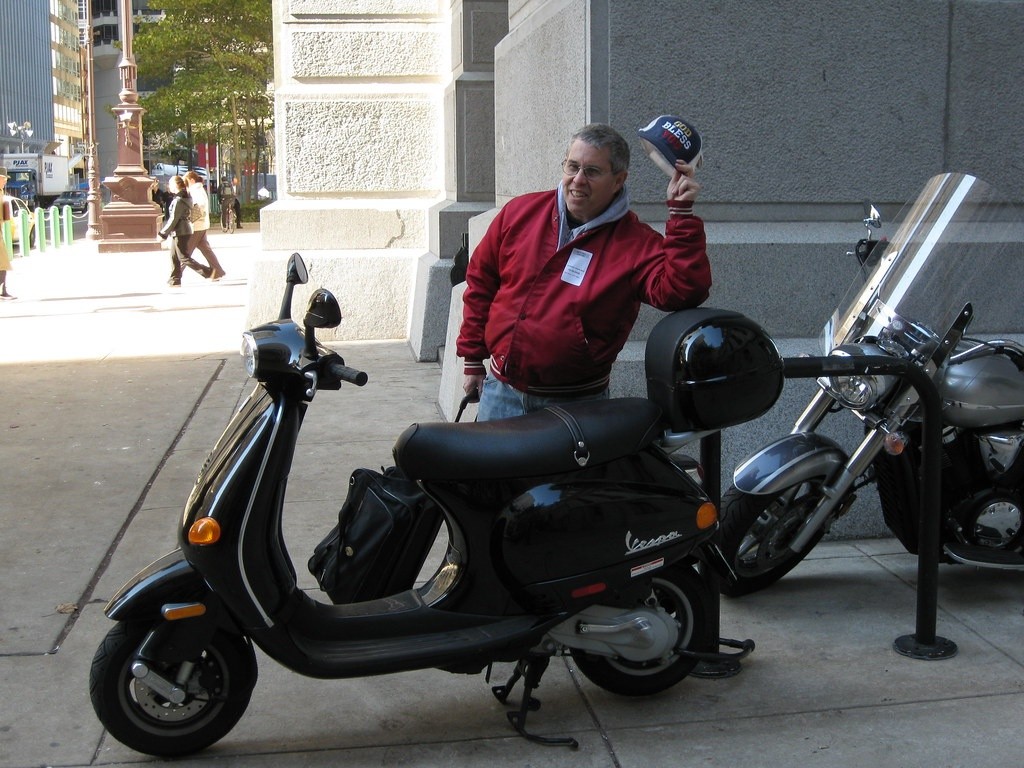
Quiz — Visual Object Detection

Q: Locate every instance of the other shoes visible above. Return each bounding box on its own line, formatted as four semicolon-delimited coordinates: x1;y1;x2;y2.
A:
0;294;16;300
168;276;181;286
213;275;225;281
207;268;212;277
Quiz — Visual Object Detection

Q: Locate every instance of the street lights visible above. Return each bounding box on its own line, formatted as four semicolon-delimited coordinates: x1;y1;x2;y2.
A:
6;120;34;155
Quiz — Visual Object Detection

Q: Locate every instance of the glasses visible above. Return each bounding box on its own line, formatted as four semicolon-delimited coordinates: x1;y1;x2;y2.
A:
561;159;616;180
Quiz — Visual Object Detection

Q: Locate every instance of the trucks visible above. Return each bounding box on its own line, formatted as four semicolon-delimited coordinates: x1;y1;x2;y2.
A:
3;154;71;211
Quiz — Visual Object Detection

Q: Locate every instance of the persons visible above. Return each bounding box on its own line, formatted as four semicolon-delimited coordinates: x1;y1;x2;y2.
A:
152;172;244;287
457;122;710;422
0;167;18;300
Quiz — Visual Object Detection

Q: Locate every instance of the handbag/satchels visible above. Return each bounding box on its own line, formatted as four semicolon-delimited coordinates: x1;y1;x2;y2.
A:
191;204;202;223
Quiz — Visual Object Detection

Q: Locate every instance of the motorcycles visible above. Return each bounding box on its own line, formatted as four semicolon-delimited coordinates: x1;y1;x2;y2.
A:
715;164;1020;603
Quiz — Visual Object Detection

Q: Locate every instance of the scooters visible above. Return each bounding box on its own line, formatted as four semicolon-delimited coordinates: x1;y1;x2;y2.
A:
86;252;783;757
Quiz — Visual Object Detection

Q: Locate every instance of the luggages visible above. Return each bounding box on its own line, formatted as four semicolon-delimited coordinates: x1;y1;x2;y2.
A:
308;390;481;605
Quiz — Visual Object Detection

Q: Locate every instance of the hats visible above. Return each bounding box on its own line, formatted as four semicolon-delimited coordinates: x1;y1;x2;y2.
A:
639;115;704;177
0;166;9;178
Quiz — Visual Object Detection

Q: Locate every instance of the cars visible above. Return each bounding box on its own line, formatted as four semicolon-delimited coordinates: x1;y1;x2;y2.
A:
52;188;87;215
2;194;38;249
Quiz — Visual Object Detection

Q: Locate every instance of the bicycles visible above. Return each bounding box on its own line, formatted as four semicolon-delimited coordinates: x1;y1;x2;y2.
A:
218;197;235;234
